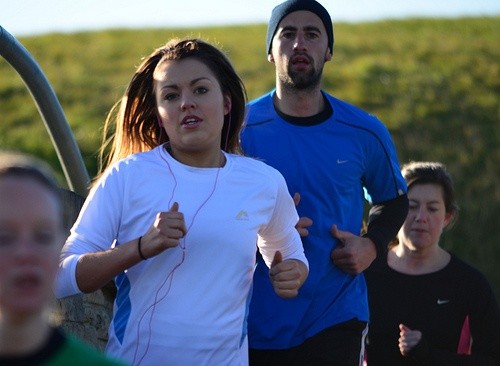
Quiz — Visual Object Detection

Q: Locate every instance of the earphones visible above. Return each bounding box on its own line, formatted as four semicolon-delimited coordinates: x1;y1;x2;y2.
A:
224;101;230;113
157;114;162;128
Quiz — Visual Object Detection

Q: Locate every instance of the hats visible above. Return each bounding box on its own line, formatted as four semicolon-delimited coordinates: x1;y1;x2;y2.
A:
267;0;334;56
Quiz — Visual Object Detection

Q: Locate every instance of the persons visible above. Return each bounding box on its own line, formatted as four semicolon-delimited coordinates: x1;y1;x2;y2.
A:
360;163;500;366
0;165;132;366
238;1;409;366
54;38;309;366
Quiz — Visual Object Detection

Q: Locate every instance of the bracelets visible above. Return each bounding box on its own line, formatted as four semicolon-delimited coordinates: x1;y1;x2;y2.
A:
137;236;146;260
